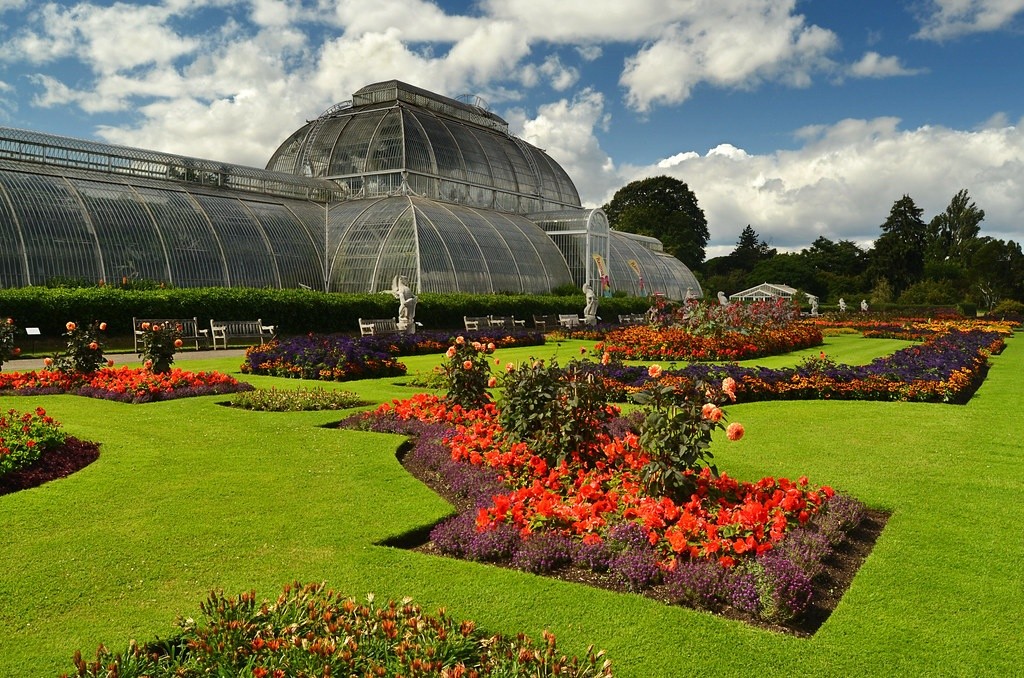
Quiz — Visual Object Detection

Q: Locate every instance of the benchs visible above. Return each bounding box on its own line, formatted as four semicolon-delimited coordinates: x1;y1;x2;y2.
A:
359;316;409;337
631;313;645;322
618;313;636;323
533;313;568;332
210;318;275;350
558;314;588;328
462;315;495;330
490;313;525;327
132;317;210;353
